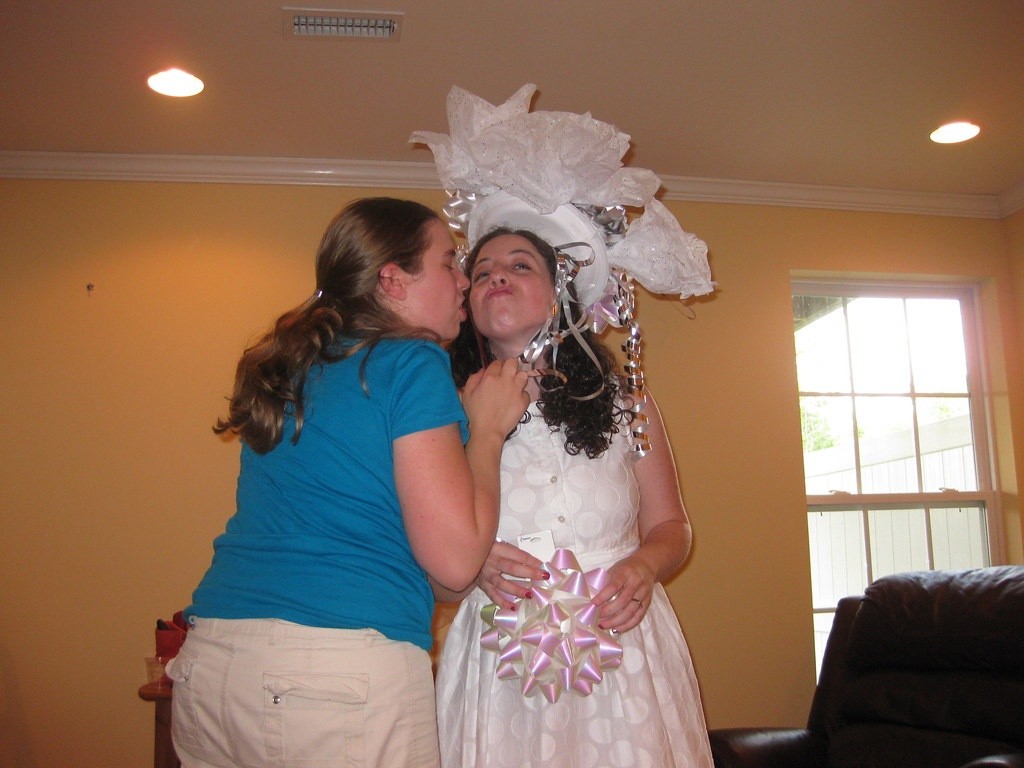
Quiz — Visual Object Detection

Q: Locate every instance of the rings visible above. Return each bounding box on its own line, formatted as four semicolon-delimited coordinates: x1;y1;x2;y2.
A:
630;598;645;608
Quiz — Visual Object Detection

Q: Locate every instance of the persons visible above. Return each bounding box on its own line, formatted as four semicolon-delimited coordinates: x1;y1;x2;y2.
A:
164;196;531;768
435;226;716;768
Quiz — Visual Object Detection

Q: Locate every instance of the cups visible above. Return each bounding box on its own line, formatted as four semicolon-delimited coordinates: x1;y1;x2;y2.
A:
144;656;173;690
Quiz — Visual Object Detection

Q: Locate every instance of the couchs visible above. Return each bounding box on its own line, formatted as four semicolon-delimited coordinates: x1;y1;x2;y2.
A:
708;565;1024;768
